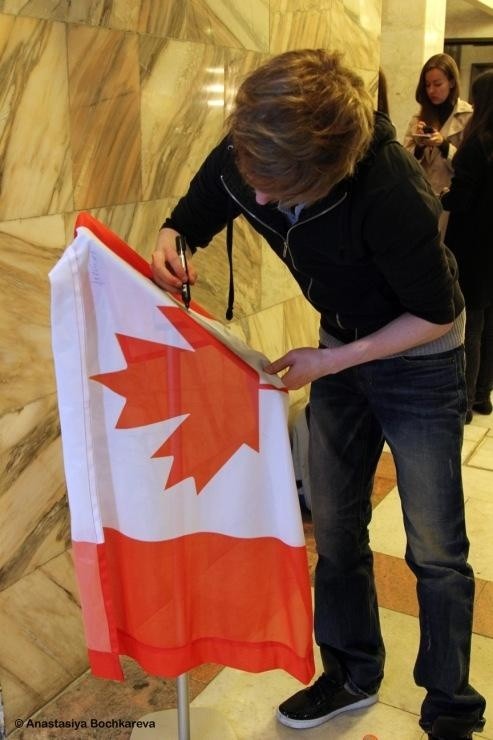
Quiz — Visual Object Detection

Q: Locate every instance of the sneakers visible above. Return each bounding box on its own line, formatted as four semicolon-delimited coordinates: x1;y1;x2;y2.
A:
276;671;379;729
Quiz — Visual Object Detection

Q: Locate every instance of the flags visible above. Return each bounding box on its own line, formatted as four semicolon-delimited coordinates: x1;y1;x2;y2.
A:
47;209;318;689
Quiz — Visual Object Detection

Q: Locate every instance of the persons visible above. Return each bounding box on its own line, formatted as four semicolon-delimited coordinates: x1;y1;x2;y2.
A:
439;70;493;424
150;48;487;739
403;53;475;243
374;64;445;227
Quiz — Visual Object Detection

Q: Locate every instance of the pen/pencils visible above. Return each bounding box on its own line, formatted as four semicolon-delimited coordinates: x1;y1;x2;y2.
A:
175;236;191;308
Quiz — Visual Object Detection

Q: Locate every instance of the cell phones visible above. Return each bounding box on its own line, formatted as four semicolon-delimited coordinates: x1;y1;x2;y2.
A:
422;125;433;134
